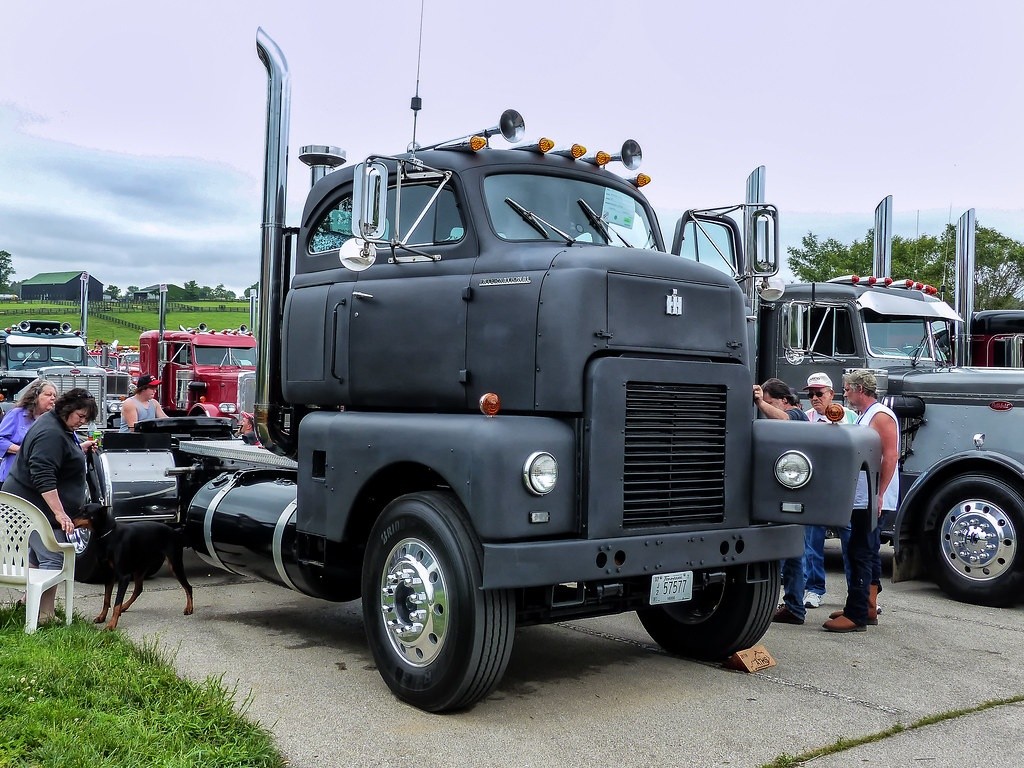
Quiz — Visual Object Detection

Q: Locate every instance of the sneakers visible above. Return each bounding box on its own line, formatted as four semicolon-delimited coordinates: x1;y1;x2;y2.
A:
876;603;882;614
803;589;821;608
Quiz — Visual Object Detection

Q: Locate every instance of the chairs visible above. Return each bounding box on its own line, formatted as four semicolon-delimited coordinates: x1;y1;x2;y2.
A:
0;490;76;634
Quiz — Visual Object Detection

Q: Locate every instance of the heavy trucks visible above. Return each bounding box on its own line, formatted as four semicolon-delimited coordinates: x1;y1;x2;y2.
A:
669;163;1024;609
0;271;106;436
58;27;883;716
89;281;258;418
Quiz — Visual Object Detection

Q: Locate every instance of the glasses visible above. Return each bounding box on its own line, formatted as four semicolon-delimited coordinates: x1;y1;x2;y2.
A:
76;391;92;398
843;389;850;392
807;389;830;398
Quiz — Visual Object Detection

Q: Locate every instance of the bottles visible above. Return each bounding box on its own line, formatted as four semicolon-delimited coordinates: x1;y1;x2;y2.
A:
93;431;102;446
86;420;97;441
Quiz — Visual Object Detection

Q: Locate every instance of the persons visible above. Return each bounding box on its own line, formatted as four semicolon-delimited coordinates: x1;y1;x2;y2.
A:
239;410;262;446
752;370;901;633
0;378;59;489
1;388;98;626
118;374;170;434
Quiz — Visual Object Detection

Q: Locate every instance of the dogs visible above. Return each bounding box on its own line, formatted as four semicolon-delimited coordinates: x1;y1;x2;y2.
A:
71;498;193;632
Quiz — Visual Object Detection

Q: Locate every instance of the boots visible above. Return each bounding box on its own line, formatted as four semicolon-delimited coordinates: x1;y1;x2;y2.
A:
822;615;867;632
829;585;878;625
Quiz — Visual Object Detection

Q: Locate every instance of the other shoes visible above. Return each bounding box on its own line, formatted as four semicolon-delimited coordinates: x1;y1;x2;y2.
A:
771;604;804;625
14;599;25;612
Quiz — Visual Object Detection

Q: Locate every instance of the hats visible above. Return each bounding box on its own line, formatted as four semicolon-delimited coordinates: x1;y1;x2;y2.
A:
137;375;162;388
850;371;878;393
802;372;833;391
